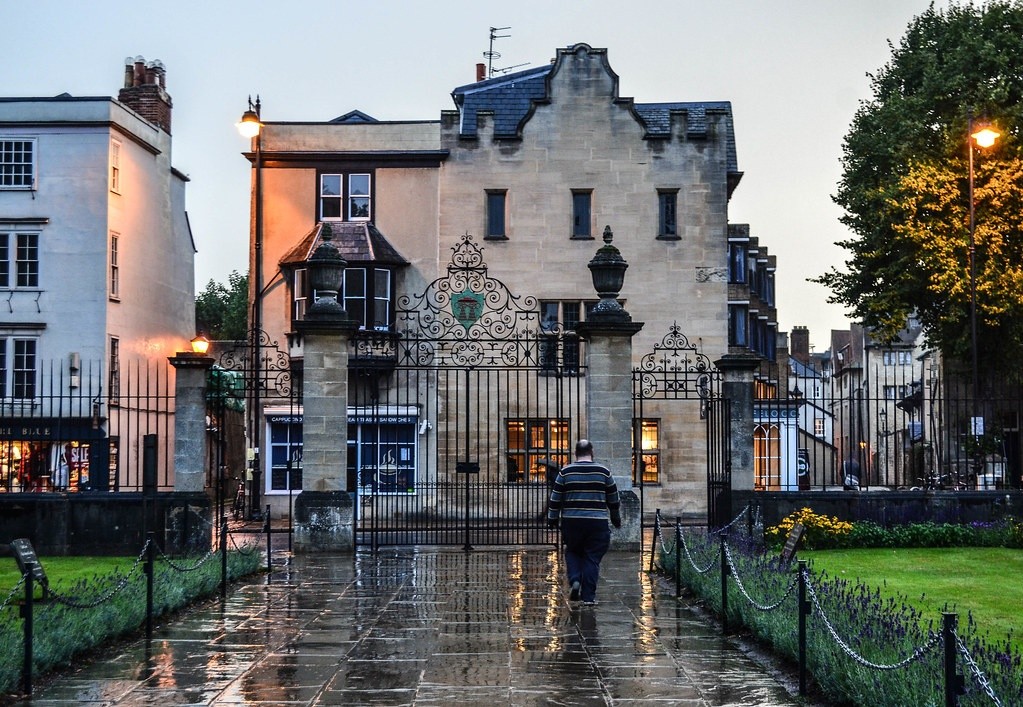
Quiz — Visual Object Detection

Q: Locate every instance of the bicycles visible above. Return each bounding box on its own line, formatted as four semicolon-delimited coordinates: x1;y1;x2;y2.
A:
230;476;244;521
909;470;967;491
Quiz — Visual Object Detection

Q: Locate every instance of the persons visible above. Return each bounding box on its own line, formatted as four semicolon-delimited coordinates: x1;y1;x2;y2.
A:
547;440;620;606
28;442;44;460
840;450;861;491
508;455;517;481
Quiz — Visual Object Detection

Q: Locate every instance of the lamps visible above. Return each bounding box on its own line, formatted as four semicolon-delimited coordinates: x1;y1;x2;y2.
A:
880;408;886;433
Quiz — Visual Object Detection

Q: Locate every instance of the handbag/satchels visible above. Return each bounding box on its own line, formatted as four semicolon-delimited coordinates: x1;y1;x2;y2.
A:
845;474;859;490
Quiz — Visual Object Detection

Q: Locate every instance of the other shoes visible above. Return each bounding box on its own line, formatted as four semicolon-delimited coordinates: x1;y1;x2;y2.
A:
568;580;581;601
583;600;599;606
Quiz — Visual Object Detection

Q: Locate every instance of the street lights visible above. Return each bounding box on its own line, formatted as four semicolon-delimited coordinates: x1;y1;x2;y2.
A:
236;92;265;525
967;107;1002;476
